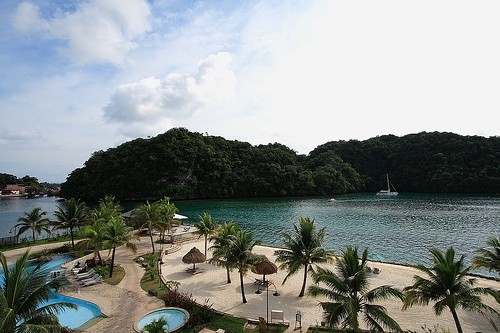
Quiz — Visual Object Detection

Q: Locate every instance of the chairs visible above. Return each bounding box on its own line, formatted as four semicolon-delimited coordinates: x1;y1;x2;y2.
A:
78;270;95;280
81;273;99;284
72;261;87;275
77;269;94;277
83;275;104;287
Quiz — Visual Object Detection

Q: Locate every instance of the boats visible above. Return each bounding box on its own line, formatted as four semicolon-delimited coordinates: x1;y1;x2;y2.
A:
375;175;399;197
